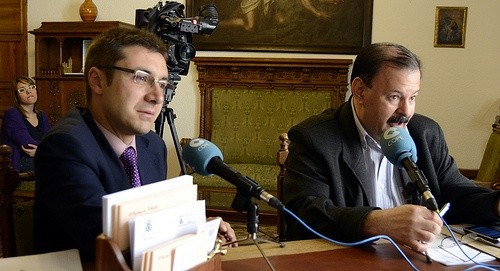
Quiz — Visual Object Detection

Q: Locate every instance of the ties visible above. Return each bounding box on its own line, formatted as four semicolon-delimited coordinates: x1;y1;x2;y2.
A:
121;147;142;188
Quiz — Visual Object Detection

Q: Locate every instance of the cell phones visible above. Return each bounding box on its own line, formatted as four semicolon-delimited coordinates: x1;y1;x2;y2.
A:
463;225;500;242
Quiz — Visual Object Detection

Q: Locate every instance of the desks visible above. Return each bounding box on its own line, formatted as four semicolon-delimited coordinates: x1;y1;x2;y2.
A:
221;223;500;271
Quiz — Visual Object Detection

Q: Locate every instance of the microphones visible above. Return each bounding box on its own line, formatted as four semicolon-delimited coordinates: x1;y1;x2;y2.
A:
184;138;282;206
381;126;439;210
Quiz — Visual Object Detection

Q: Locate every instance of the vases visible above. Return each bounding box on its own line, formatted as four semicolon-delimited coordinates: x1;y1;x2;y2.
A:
79;0;98;21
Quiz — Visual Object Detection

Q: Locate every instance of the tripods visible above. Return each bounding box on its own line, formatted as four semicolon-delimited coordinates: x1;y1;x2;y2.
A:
222;235;285;271
156;108;189;175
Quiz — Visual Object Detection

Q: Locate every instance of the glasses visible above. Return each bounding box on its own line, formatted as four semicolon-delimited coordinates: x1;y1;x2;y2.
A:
86;65;168;90
15;85;37;93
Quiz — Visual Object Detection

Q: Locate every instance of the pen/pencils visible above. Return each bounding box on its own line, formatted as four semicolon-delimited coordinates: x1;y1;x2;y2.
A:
419;203;451;244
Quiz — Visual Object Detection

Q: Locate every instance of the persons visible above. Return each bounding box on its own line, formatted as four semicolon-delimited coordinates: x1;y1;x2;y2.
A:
4;76;50;174
31;26;239;271
280;42;500;251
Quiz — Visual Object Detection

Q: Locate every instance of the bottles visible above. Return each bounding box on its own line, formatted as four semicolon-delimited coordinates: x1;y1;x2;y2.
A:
78;0;98;21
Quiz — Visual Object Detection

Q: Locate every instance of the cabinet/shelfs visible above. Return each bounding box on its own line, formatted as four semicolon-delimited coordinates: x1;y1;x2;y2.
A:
26;21;135;129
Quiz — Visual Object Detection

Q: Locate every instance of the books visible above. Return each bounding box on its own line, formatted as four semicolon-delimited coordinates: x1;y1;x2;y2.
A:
404;224;500;267
102;174;223;271
0;248;85;271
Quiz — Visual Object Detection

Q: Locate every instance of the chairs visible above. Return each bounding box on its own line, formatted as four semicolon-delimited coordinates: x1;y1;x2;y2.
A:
0;144;34;258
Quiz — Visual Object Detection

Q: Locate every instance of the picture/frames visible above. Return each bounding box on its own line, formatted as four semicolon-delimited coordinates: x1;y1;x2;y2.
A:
434;6;467;48
184;0;374;55
